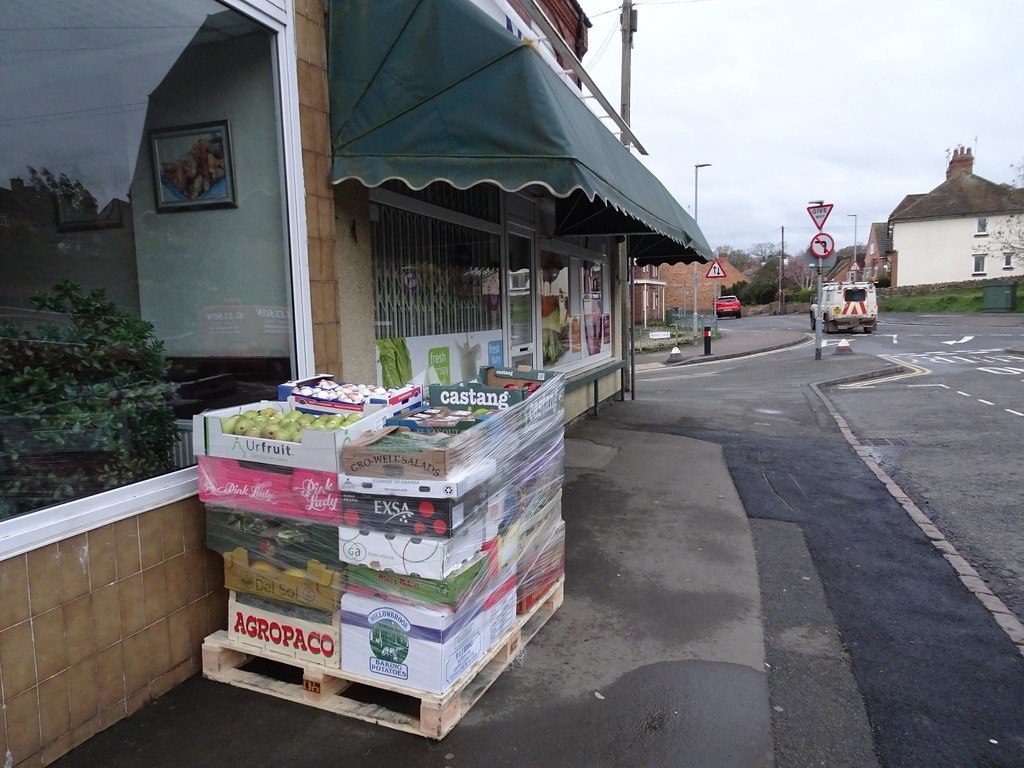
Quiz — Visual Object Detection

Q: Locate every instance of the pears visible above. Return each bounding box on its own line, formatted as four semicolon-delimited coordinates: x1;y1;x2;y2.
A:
221;403;361;444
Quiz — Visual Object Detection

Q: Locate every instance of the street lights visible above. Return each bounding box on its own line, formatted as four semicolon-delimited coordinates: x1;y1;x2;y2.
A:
693;164;718;344
847;214;857;263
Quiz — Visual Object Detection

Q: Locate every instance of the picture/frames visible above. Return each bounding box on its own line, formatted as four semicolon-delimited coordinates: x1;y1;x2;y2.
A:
147;118;239;214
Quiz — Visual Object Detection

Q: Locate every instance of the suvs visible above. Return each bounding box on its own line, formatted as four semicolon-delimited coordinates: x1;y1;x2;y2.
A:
713;295;742;319
808;280;878;336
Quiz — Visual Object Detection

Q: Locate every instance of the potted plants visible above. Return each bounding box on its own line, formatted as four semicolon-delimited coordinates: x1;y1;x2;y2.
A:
50;159;125;234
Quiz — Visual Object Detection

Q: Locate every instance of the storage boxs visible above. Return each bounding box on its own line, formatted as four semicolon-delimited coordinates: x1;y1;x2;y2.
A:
192;366;567;732
